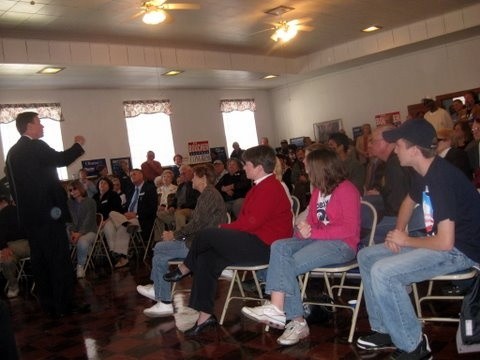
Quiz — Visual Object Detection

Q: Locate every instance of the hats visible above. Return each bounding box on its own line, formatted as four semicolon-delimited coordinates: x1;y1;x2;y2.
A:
383;119;438;148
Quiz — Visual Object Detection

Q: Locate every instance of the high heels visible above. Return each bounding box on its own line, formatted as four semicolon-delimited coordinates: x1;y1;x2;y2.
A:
185;315;220;336
163;267;193;282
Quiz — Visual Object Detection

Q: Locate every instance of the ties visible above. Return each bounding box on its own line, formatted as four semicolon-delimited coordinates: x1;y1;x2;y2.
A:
130;188;139;212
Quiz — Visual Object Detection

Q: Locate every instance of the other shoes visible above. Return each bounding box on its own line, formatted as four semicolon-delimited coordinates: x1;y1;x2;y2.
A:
113;260;130;270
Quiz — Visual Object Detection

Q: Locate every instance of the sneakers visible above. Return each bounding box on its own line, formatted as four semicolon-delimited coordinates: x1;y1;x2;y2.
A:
137;285;156;300
76;264;85;278
8;284;19;297
277;319;310;345
383;334;434;360
242;300;286;329
143;302;174;318
358;332;397;350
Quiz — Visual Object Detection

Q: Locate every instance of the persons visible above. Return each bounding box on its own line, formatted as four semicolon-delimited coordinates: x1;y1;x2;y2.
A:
214;143;252;217
4;112;91;318
273;137;311;214
422;90;480;193
173;154;183;170
137;163;227;316
242;148;361;345
141;150;162;185
79;161;201;269
261;137;268;145
242;143;329;309
66;181;98;276
0;168;31;297
357;118;480;360
163;145;293;336
328;123;427;249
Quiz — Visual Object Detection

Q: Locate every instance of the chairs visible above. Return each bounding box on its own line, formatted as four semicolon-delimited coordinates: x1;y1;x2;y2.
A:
4;183;480;352
